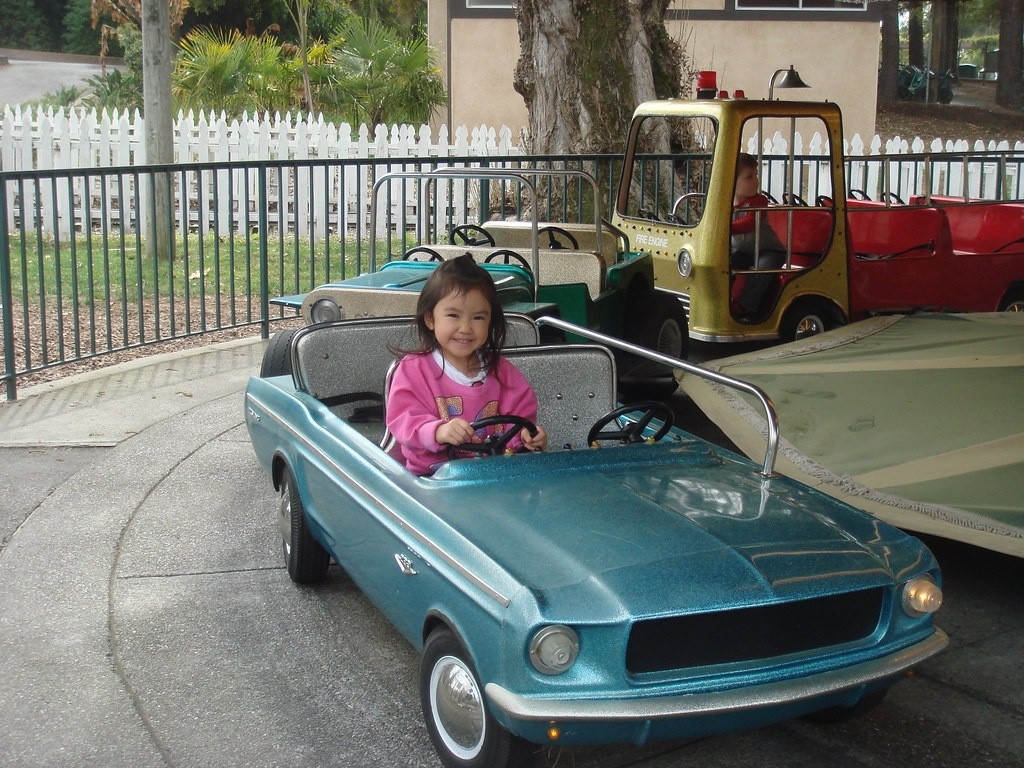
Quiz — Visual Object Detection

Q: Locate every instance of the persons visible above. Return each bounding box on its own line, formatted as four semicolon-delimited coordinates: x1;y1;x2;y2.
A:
730;152;787;323
386;257;548;478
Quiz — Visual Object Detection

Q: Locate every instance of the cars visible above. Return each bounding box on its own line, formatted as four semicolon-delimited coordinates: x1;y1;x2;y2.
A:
298;167;692;404
241;308;951;768
612;62;1024;344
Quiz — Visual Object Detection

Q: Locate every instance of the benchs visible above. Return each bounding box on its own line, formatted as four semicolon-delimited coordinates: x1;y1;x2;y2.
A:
291;311;542;451
379;343;620;451
767;196;1024;272
474;221;623;269
403;245;607;301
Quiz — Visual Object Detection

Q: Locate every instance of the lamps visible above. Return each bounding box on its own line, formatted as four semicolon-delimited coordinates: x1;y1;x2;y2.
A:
768;65;812;100
